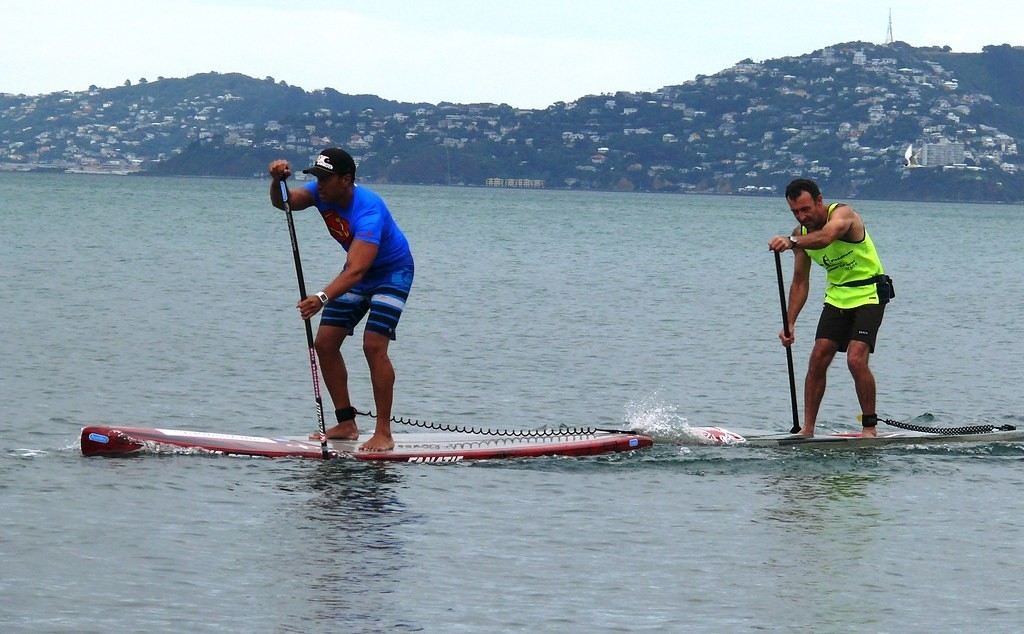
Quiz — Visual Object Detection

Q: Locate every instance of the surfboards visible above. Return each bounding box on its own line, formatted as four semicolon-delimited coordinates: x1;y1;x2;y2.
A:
607;419;1022;447
80;424;652;466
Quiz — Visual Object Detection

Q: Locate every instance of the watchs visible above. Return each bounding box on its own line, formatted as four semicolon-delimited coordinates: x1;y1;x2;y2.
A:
787;236;798;250
314;291;330;307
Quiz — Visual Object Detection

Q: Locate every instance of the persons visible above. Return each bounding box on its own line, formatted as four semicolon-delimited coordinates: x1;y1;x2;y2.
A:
269;148;415;451
768;178;886;439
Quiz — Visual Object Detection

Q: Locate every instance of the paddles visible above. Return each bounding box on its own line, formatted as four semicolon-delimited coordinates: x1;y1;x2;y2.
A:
279;173;331;458
771;252;802;433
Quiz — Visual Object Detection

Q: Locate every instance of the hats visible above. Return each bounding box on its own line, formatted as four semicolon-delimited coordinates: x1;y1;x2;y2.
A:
303;147;356;179
